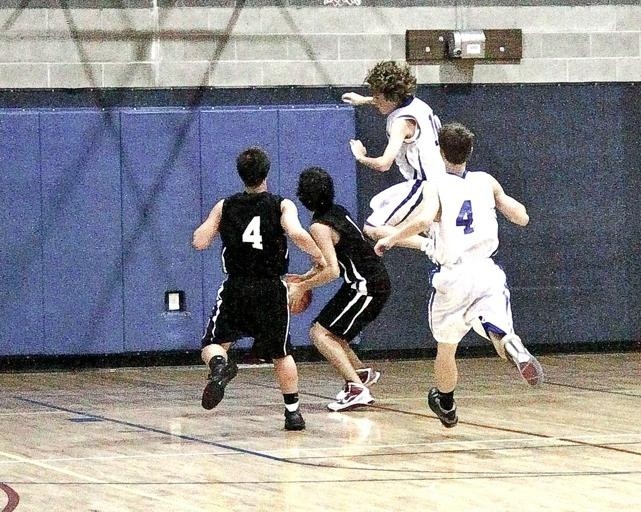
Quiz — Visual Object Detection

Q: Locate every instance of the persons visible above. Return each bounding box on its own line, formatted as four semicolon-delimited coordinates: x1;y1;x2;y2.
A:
374;120;547;430
188;142;329;431
284;165;394;413
338;58;445;253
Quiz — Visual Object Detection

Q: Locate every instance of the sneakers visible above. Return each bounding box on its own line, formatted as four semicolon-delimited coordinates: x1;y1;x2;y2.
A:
327;382;376;412
284;404;306;431
201;360;238;410
336;366;381;402
428;387;458;428
504;337;544;389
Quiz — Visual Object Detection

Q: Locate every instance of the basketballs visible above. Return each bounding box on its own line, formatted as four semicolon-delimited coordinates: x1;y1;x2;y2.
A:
287;275;311;314
